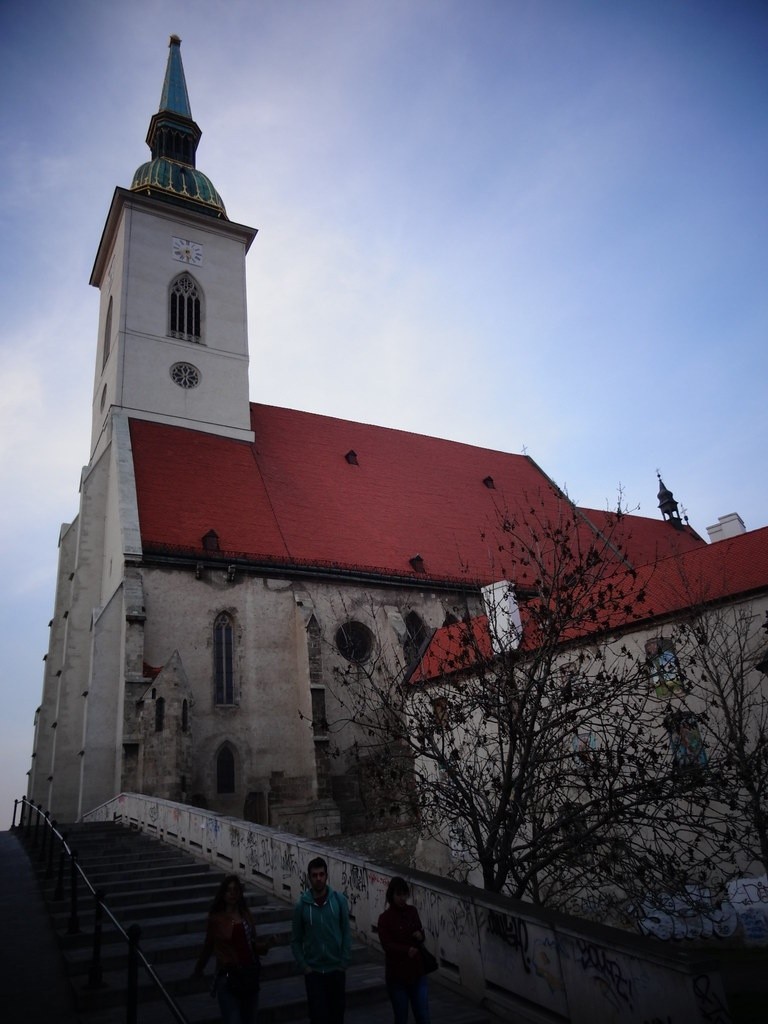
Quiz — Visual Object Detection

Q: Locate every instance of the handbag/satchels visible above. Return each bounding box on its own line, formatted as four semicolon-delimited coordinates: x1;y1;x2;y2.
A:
240;955;266;981
403;928;438;988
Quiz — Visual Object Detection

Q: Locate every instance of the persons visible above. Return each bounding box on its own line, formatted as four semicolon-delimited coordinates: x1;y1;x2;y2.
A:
204;876;278;1024
378;876;432;1024
290;856;352;1024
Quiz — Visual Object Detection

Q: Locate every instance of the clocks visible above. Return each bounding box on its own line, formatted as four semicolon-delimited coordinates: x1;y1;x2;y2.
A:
171;235;204;269
